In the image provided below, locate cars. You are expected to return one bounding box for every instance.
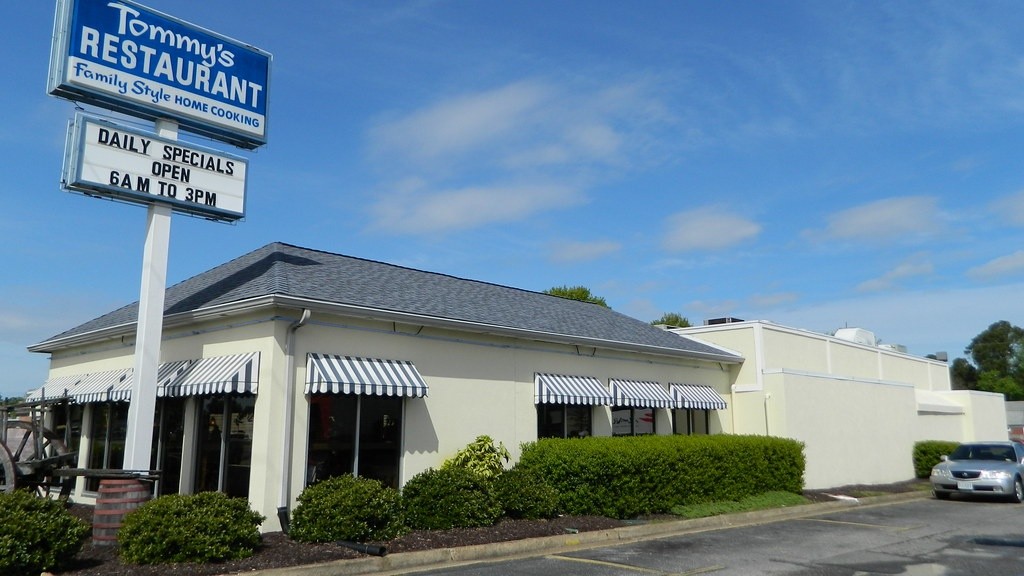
[929,441,1024,503]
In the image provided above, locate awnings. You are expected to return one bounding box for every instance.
[25,374,88,404]
[304,352,429,397]
[165,351,259,397]
[109,358,193,400]
[608,378,676,408]
[60,368,133,405]
[668,383,728,410]
[534,372,614,406]
